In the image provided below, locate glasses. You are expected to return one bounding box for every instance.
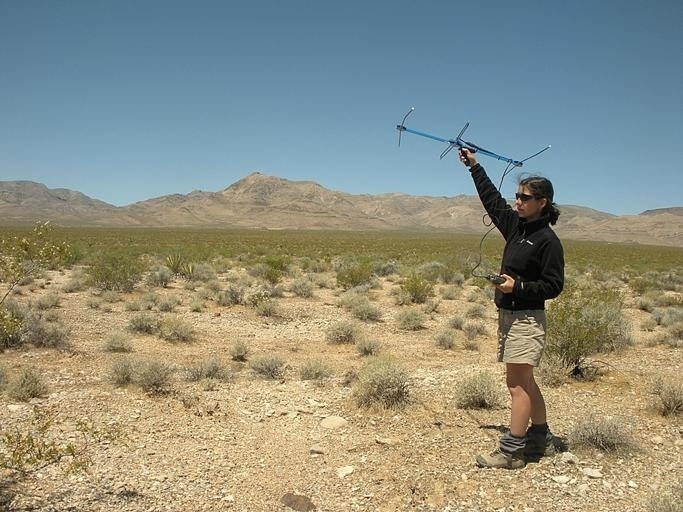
[517,193,535,202]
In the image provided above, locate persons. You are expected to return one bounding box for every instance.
[457,147,566,471]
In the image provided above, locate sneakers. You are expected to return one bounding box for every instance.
[523,432,555,455]
[477,447,525,469]
